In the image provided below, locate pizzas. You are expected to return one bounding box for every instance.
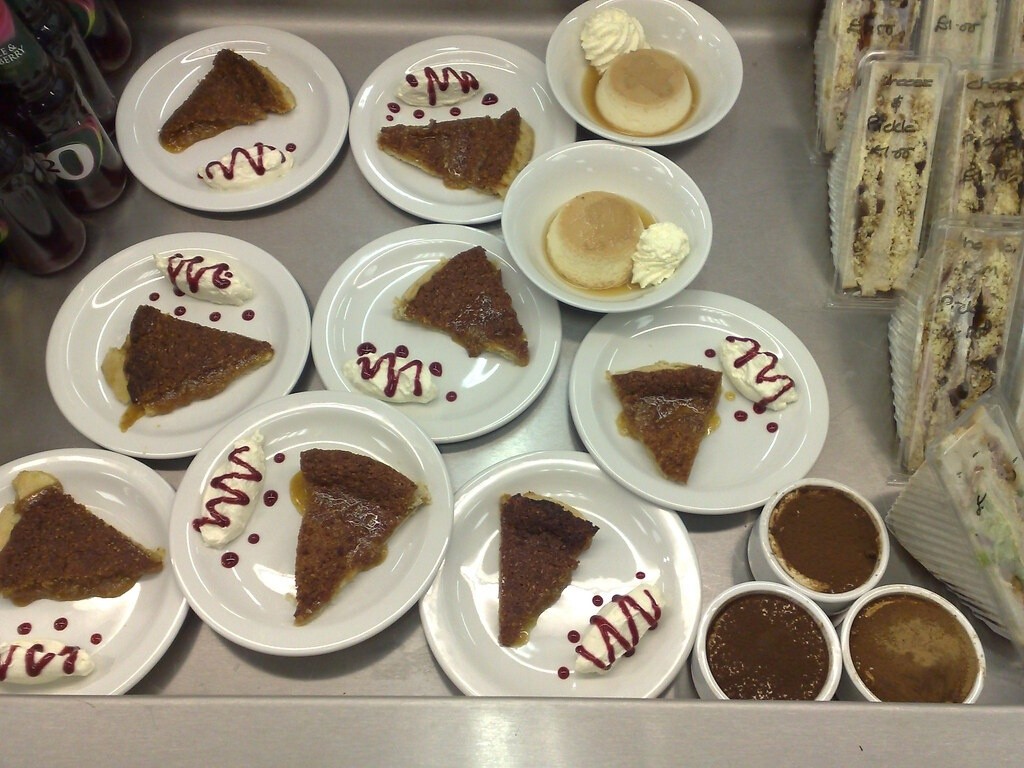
[605,359,724,487]
[494,491,601,648]
[376,106,537,200]
[101,304,274,417]
[159,50,298,154]
[292,448,432,626]
[0,469,166,604]
[394,243,529,366]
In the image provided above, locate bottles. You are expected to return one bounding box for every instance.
[0,0,132,277]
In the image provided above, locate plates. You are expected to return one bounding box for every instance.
[43,229,312,461]
[418,449,704,700]
[349,34,577,225]
[114,23,350,214]
[567,289,830,517]
[168,389,456,657]
[0,447,191,697]
[311,222,563,447]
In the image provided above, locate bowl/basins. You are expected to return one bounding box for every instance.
[744,476,891,627]
[499,139,714,315]
[544,1,745,147]
[689,578,842,704]
[841,583,987,705]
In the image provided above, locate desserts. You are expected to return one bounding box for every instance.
[546,189,690,290]
[580,6,693,136]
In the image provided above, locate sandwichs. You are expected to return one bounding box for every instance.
[812,0,1024,653]
